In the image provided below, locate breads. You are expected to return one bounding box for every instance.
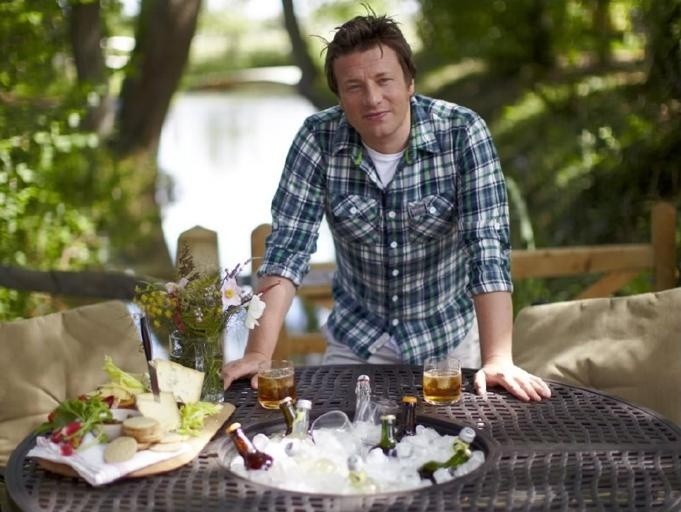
[134,359,206,430]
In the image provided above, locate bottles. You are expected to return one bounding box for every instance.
[225,423,274,471]
[354,374,480,493]
[284,442,336,482]
[279,395,312,442]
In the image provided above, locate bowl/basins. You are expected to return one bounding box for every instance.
[75,406,144,442]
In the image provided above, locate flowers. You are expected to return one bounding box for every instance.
[129,239,282,398]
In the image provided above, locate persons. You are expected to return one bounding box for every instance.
[220,2,552,402]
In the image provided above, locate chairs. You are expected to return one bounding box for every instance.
[510,287,681,430]
[1,301,158,480]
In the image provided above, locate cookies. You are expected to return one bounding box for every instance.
[104,415,180,464]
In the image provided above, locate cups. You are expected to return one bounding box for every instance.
[422,356,464,406]
[257,358,297,411]
[310,410,363,454]
